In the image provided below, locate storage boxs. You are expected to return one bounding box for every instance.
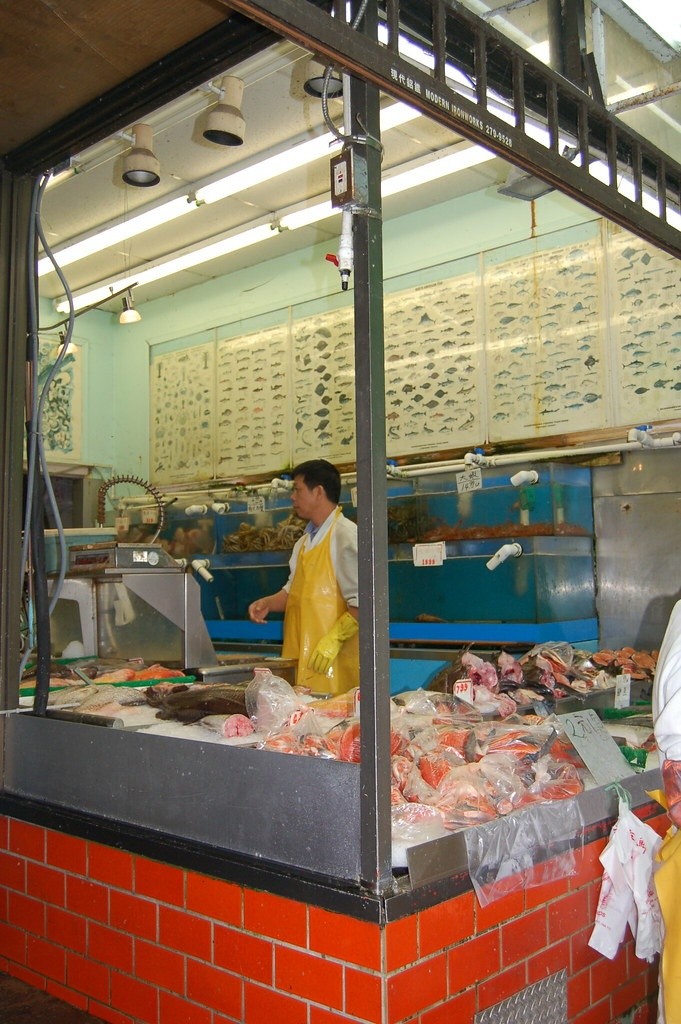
[44,528,118,574]
[152,462,600,648]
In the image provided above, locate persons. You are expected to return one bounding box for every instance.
[249,460,360,700]
[652,598,681,1024]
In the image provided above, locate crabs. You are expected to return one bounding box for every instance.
[222,513,307,551]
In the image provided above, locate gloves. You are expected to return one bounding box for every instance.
[307,611,359,675]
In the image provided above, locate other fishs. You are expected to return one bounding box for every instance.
[20,638,657,841]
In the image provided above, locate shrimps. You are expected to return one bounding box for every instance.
[422,516,588,542]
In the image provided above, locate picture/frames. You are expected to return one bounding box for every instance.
[22,337,84,460]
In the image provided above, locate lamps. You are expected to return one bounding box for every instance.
[114,124,160,187]
[57,322,78,355]
[53,140,497,314]
[498,145,599,202]
[119,289,141,324]
[331,0,681,232]
[37,98,422,276]
[303,54,343,98]
[199,76,247,145]
